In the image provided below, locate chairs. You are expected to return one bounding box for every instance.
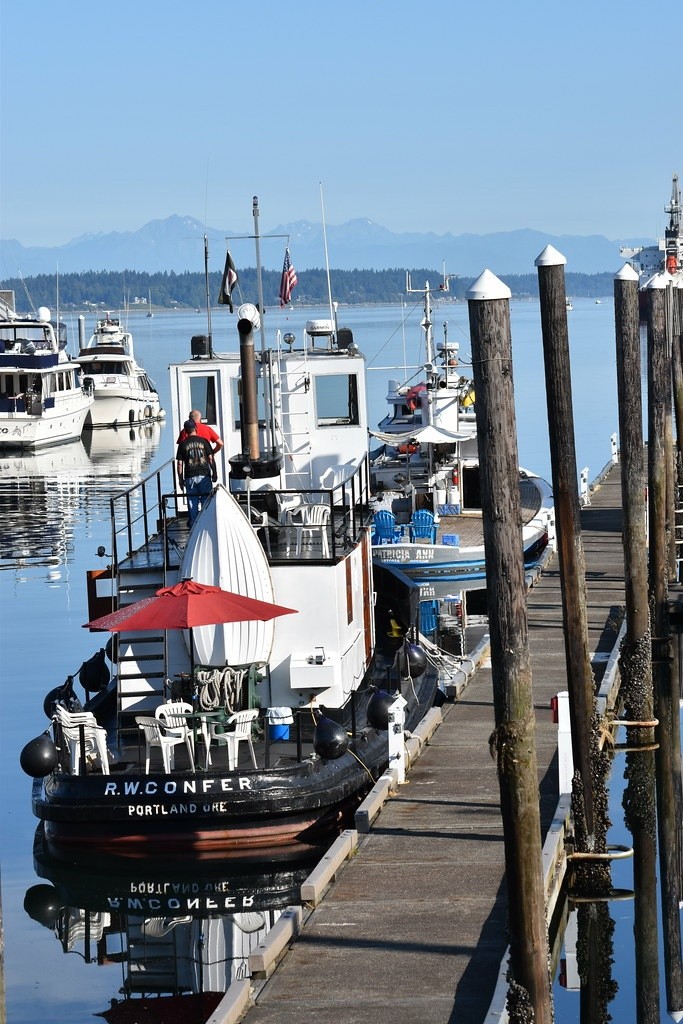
[56,704,111,775]
[285,503,331,559]
[205,708,258,772]
[155,702,213,769]
[319,463,359,521]
[240,504,273,559]
[407,508,440,544]
[135,716,196,774]
[419,600,437,635]
[266,483,314,538]
[370,509,405,545]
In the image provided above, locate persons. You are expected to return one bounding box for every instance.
[176,410,223,464]
[174,420,219,530]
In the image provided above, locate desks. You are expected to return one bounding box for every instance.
[167,711,220,772]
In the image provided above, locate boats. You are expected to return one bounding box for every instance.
[32,179,466,858]
[357,257,557,656]
[78,418,160,465]
[0,260,94,452]
[66,273,166,429]
[27,814,358,1024]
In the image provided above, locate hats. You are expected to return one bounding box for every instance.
[184,420,196,428]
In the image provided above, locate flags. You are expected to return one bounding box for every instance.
[278,248,297,308]
[217,250,239,313]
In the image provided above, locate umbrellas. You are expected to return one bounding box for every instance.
[81,576,299,767]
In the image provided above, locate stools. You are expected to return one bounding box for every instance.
[442,533,459,546]
